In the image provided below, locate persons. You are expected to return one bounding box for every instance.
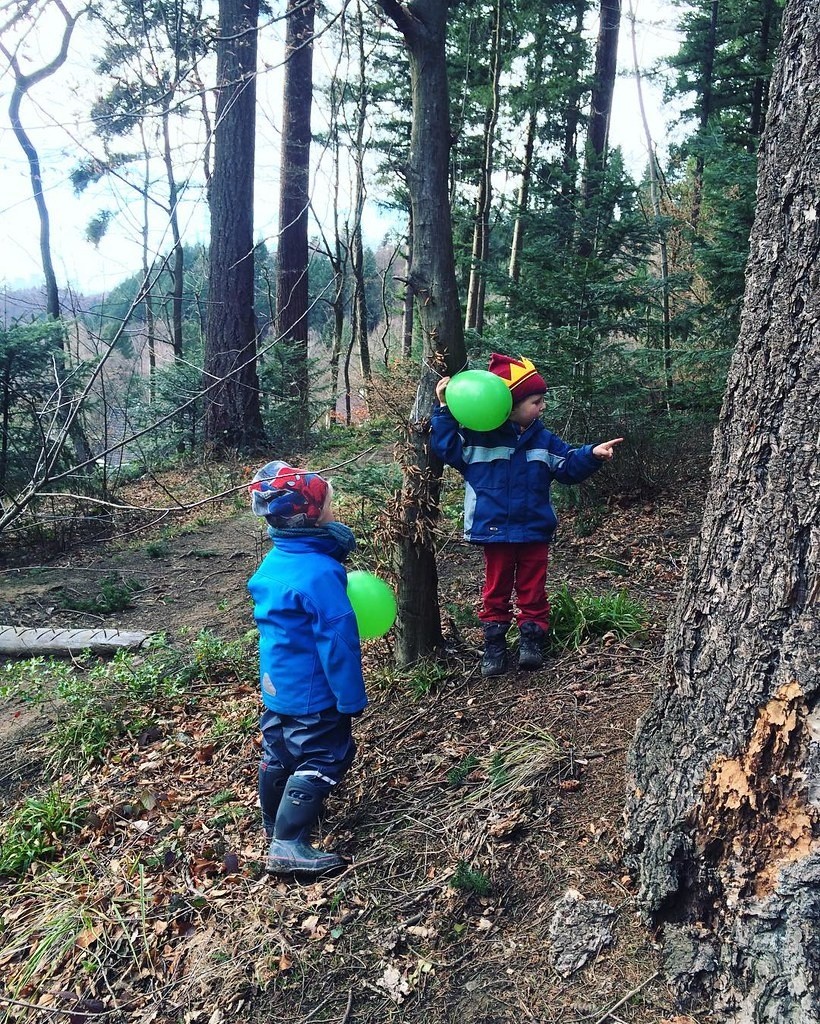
[429,353,624,677]
[247,460,368,874]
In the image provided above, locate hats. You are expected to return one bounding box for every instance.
[248,461,328,528]
[488,354,547,403]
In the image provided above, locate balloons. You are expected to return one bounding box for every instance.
[346,571,397,637]
[445,370,512,431]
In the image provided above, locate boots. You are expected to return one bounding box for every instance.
[481,622,511,676]
[264,775,348,877]
[259,761,292,844]
[518,621,545,669]
